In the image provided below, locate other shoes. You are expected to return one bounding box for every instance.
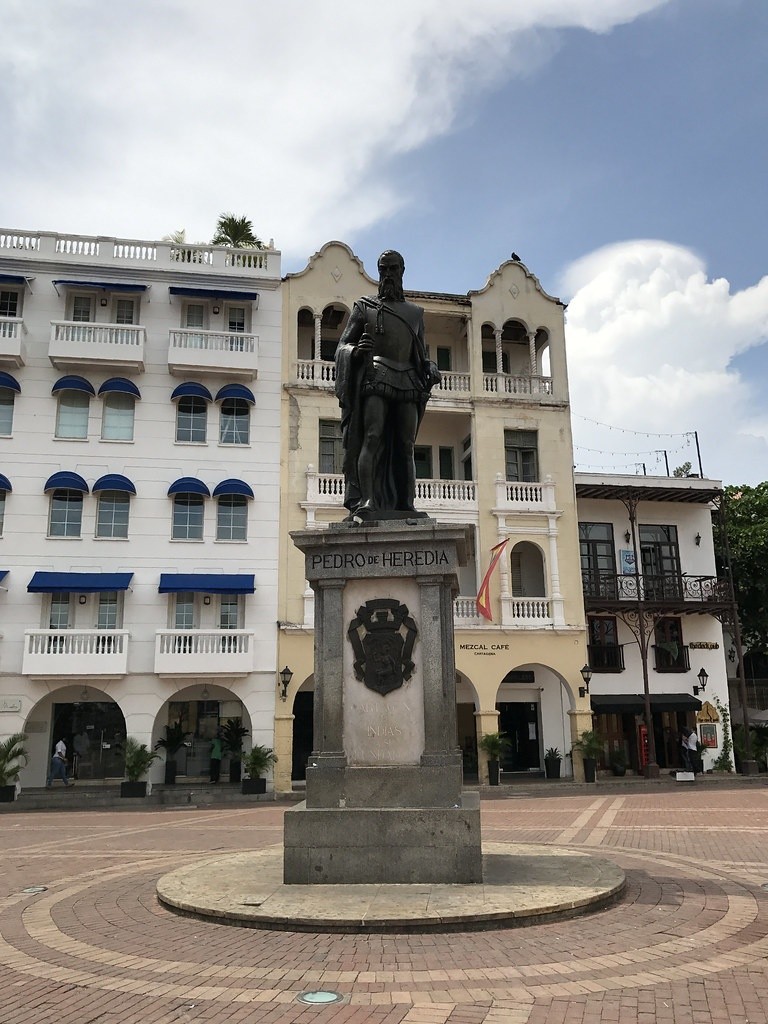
[47,782,51,789]
[65,783,75,787]
[208,779,216,784]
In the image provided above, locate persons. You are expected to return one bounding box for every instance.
[207,733,224,784]
[335,251,442,523]
[46,735,75,789]
[683,727,698,775]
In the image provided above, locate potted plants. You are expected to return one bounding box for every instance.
[480,731,513,784]
[611,750,627,776]
[118,738,164,798]
[156,721,192,784]
[0,731,30,801]
[239,744,278,794]
[571,727,606,782]
[544,747,563,778]
[217,716,252,782]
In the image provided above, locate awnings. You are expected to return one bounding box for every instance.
[157,573,256,594]
[0,371,21,393]
[27,571,134,592]
[590,693,702,714]
[170,381,256,405]
[0,273,35,295]
[168,286,260,311]
[51,374,141,400]
[167,476,256,499]
[0,571,9,591]
[44,471,137,495]
[0,473,12,492]
[51,279,152,304]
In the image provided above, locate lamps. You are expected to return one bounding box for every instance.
[202,684,209,698]
[696,533,701,545]
[81,686,89,700]
[101,299,107,306]
[79,595,86,604]
[625,529,631,543]
[213,307,219,314]
[279,665,293,697]
[579,664,593,697]
[204,596,210,604]
[693,667,709,695]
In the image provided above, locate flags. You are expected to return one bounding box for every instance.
[476,538,511,621]
